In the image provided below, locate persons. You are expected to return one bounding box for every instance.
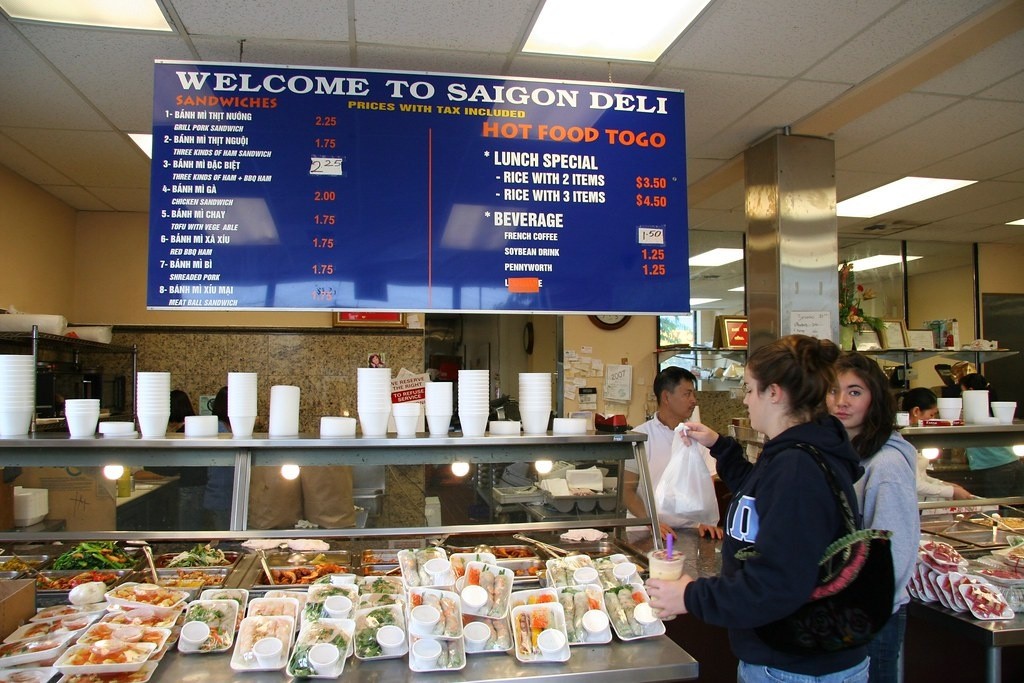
[368,354,385,368]
[645,335,867,683]
[622,366,723,541]
[959,373,1024,498]
[134,390,208,531]
[825,355,921,683]
[203,387,237,532]
[892,387,975,501]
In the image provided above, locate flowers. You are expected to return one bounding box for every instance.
[838,260,888,331]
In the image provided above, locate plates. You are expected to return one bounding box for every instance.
[908,539,1015,620]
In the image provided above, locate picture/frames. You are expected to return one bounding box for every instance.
[853,330,884,350]
[332,312,407,328]
[879,318,909,348]
[719,315,748,347]
[907,329,935,349]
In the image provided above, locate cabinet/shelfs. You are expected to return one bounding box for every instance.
[0,325,139,432]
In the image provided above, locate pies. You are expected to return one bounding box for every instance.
[401,547,646,668]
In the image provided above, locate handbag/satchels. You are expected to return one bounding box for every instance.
[654,423,720,526]
[733,445,895,652]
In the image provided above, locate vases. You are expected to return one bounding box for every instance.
[839,324,853,351]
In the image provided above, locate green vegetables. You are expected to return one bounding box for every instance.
[167,543,223,567]
[288,572,398,678]
[50,542,139,572]
[186,595,241,637]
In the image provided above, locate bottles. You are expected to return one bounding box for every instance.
[690,366,715,380]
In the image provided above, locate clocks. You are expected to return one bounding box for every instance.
[588,315,632,330]
[523,322,534,354]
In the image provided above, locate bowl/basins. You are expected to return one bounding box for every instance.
[0,355,36,436]
[137,373,171,438]
[227,372,257,439]
[519,373,552,434]
[937,390,1017,425]
[356,367,489,438]
[148,549,666,683]
[65,399,100,437]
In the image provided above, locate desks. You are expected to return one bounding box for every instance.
[115,475,180,530]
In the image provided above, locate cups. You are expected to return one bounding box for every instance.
[647,549,686,620]
